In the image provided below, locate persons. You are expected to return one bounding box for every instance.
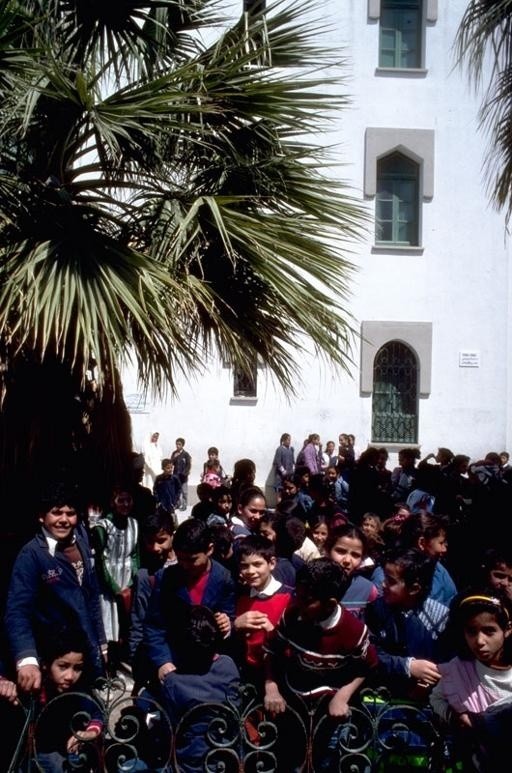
[7,488,108,691]
[265,434,354,505]
[204,447,222,476]
[91,459,457,772]
[355,444,511,509]
[429,557,511,728]
[140,431,164,494]
[171,438,191,510]
[0,642,106,771]
